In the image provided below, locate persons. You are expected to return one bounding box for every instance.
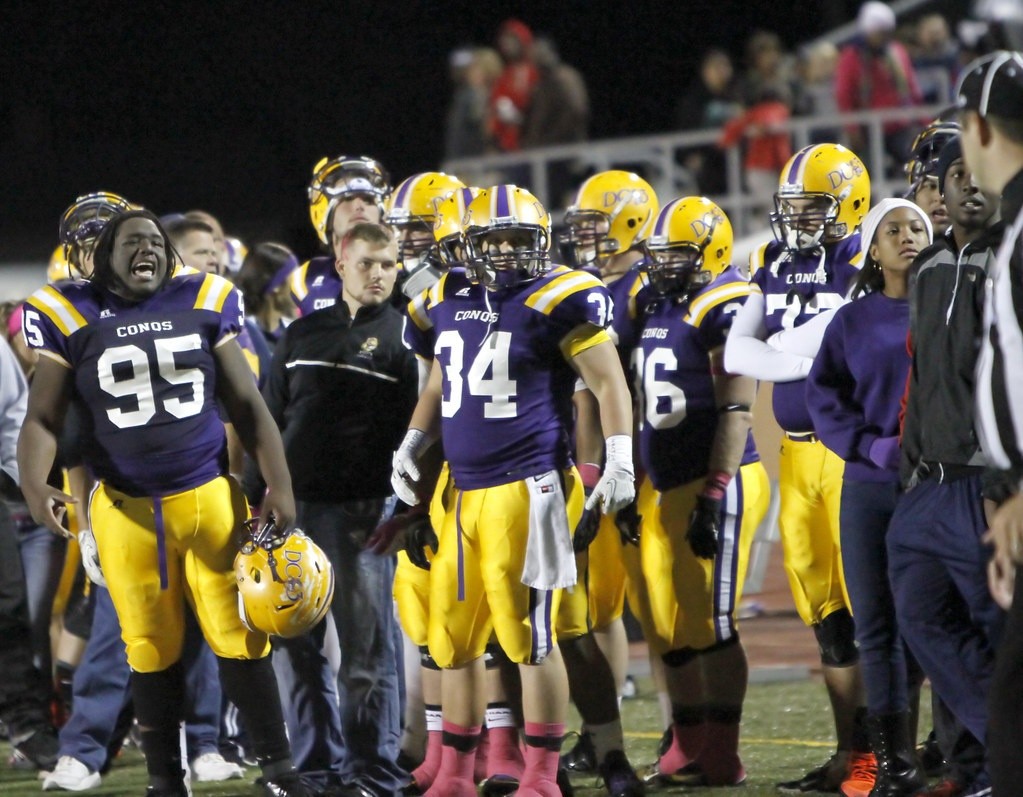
[391,185,636,796]
[621,194,760,788]
[0,0,1023,797]
[18,210,294,797]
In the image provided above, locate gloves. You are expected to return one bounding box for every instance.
[389,427,434,509]
[584,435,643,545]
[682,492,723,560]
[869,434,902,469]
[366,510,440,571]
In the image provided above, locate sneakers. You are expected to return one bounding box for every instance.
[598,748,648,797]
[774,749,880,797]
[634,724,749,791]
[189,753,245,782]
[42,756,102,791]
[558,730,606,790]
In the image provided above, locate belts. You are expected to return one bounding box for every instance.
[787,432,817,443]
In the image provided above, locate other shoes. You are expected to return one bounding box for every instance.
[257,770,308,797]
[6,730,42,771]
[318,782,373,797]
[915,741,992,797]
[146,769,194,797]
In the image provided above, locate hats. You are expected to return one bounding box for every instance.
[937,135,962,197]
[938,50,1023,120]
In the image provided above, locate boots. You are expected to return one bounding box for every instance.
[860,711,930,796]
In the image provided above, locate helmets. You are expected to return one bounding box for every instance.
[234,514,336,642]
[307,153,733,306]
[766,143,872,254]
[59,190,135,271]
[902,121,963,198]
